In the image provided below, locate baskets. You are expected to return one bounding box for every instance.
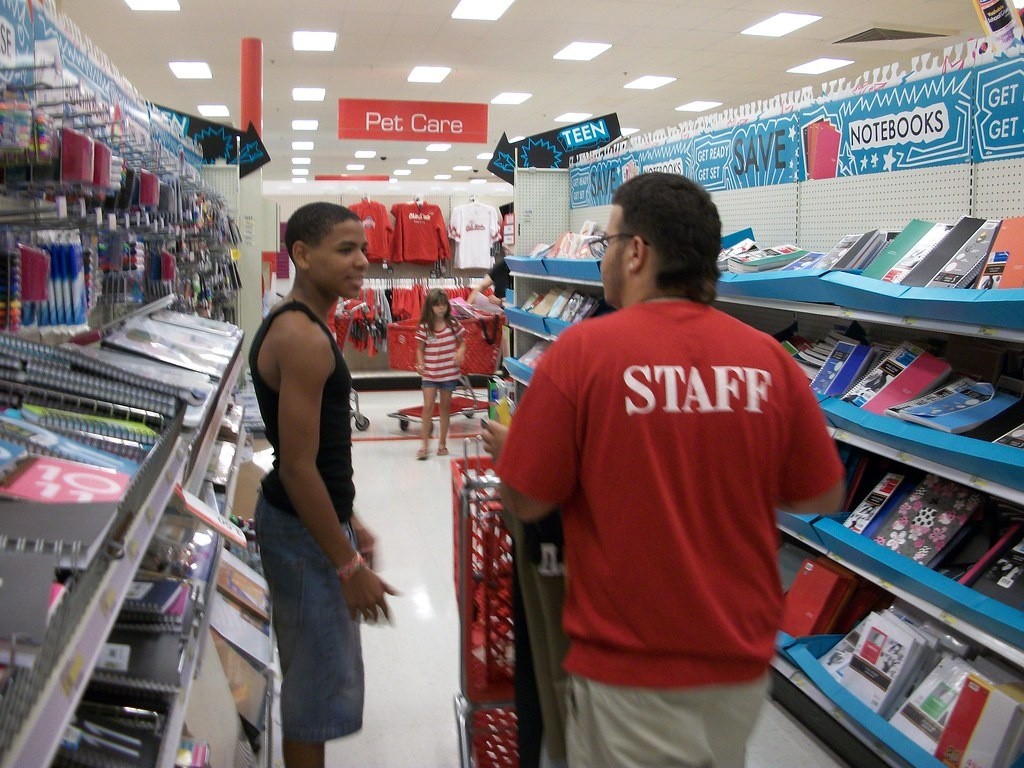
[388,313,504,374]
[449,457,512,704]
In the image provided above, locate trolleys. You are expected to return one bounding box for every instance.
[449,455,566,768]
[387,314,507,435]
[333,297,369,431]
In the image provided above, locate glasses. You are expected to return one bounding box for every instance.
[588,233,651,259]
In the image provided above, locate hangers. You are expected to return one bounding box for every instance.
[465,278,493,290]
[468,194,480,207]
[360,277,464,292]
[413,193,424,206]
[361,193,370,204]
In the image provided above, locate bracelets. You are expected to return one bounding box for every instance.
[336,553,363,578]
[418,364,424,365]
[501,299,502,306]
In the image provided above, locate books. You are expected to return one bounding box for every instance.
[0,352,214,470]
[779,321,1024,447]
[517,220,608,372]
[717,214,1024,290]
[52,580,194,768]
[0,501,117,669]
[773,456,1024,768]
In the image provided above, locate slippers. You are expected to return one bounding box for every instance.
[437,447,447,456]
[416,451,427,460]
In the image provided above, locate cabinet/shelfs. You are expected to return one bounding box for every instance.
[0,0,284,768]
[503,30,1024,768]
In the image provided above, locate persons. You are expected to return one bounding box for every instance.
[482,171,844,768]
[415,288,467,458]
[248,202,400,768]
[468,258,514,377]
